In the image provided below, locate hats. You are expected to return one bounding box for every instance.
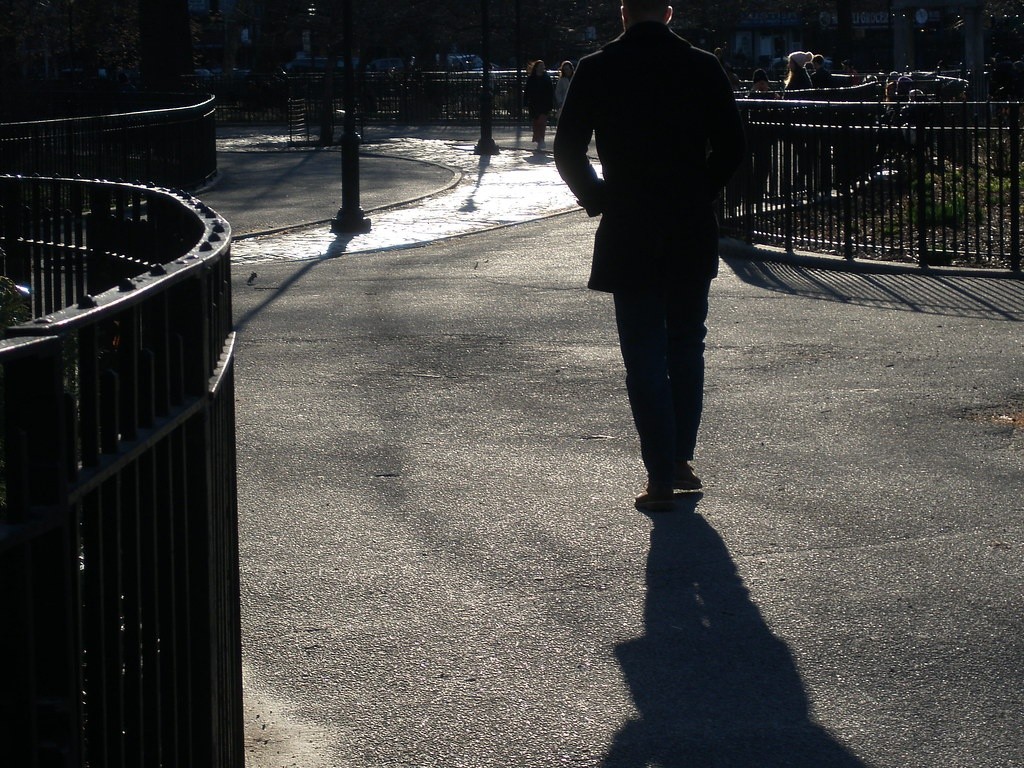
[753,69,768,83]
[791,52,812,68]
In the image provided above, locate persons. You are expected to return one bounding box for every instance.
[555,0,741,512]
[523,48,1024,153]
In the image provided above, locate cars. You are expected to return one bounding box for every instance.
[292,54,507,72]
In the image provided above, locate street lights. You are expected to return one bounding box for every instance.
[308,2,315,79]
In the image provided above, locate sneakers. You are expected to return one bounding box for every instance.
[636,493,675,508]
[672,465,703,490]
